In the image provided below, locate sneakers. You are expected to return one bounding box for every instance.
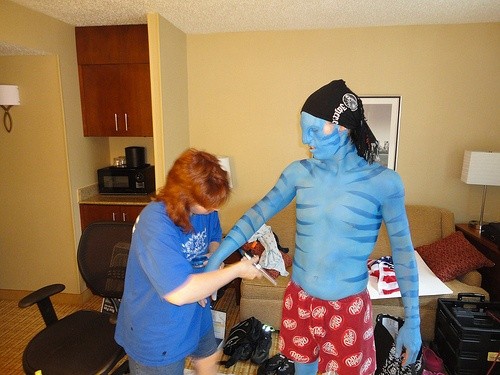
[238,334,272,365]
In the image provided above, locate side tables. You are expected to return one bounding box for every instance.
[456,223,500,324]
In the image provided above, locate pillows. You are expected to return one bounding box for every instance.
[242,242,292,279]
[414,229,494,283]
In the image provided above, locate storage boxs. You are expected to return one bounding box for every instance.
[433,292,500,375]
[210,309,227,351]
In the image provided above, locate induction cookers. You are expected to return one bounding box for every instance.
[97,166,155,196]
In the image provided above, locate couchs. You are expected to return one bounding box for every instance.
[242,199,489,343]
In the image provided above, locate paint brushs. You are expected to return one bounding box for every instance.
[239,248,278,286]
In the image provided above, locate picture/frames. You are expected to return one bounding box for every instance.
[312,95,403,173]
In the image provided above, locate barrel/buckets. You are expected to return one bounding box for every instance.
[124,145,145,168]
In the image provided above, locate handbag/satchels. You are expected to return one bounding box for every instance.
[373,313,424,375]
[218,316,264,369]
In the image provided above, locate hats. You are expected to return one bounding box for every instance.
[300,79,383,166]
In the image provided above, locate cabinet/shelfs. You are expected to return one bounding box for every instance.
[78,204,146,281]
[78,64,153,138]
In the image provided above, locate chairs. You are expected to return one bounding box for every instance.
[18,222,135,375]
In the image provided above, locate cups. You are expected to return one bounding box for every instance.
[112,156,126,168]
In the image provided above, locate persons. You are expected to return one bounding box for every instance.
[114,148,263,375]
[197,78,423,375]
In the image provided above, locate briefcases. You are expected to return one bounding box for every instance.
[433,292,500,375]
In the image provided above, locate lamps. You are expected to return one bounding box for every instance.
[0,85,21,133]
[215,156,232,189]
[460,150,500,230]
